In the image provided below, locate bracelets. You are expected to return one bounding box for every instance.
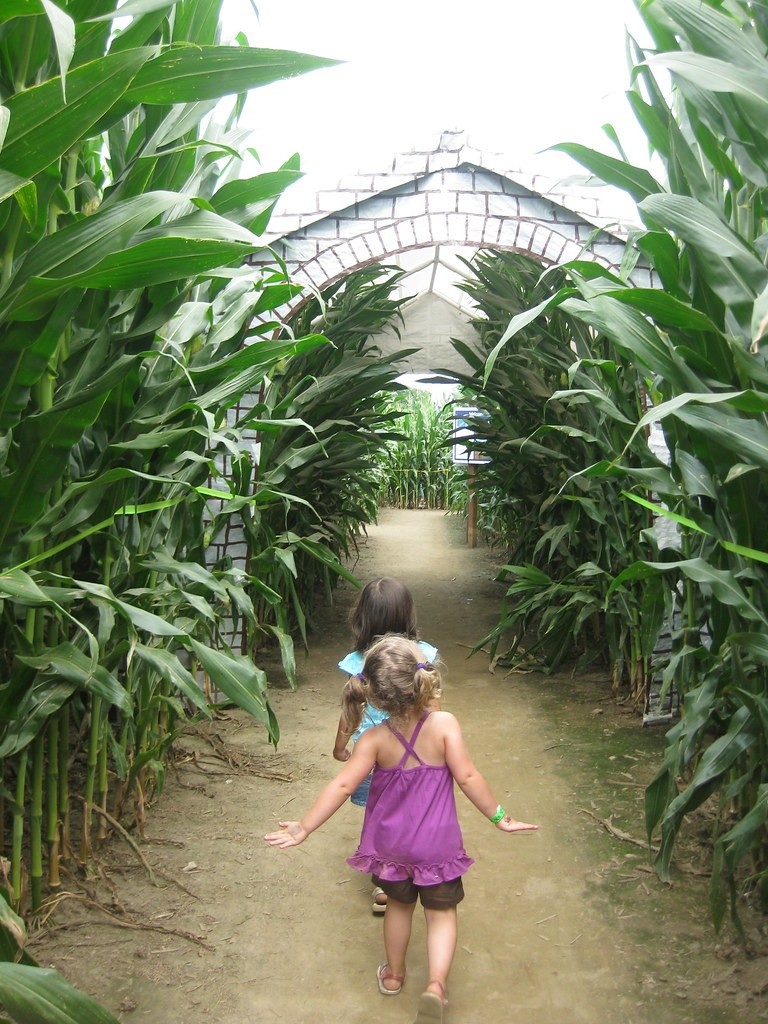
[490,806,506,823]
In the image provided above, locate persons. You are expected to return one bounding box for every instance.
[333,578,438,912]
[263,638,539,1024]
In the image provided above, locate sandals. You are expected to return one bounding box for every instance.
[413,980,448,1024]
[371,887,388,912]
[377,962,406,995]
